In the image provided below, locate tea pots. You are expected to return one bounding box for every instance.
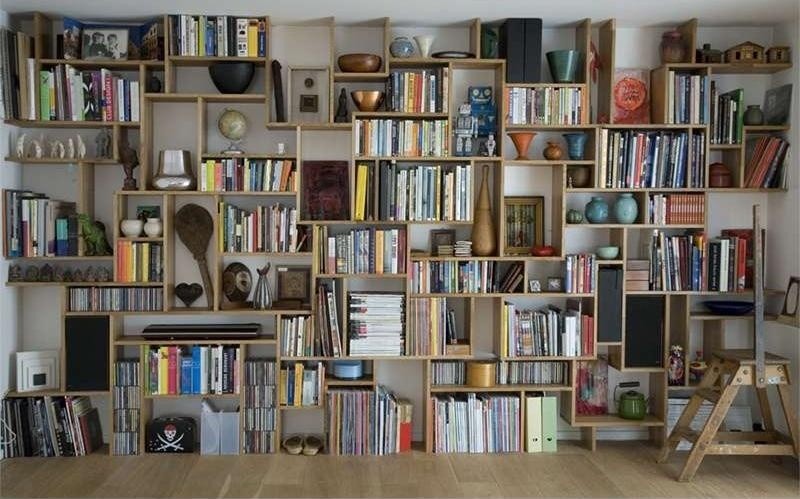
[613,380,656,420]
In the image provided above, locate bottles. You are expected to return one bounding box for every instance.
[253,262,274,311]
[743,104,763,125]
[389,37,411,58]
[144,218,162,239]
[658,32,686,63]
[586,196,609,224]
[542,142,561,160]
[612,193,640,224]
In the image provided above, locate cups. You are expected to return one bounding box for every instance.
[506,133,539,161]
[562,134,589,161]
[413,33,434,58]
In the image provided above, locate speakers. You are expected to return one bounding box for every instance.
[627,295,664,368]
[64,315,108,391]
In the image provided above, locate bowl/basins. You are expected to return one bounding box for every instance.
[209,62,255,95]
[120,220,144,238]
[596,246,620,260]
[337,54,382,72]
[351,91,386,112]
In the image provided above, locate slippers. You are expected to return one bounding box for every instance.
[282,434,323,456]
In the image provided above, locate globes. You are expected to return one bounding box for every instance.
[214,107,248,154]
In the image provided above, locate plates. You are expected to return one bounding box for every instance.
[702,300,754,316]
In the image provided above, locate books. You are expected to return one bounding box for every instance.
[502,19,794,452]
[316,66,519,456]
[2,13,324,452]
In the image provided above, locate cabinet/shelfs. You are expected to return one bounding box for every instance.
[0,9,793,456]
